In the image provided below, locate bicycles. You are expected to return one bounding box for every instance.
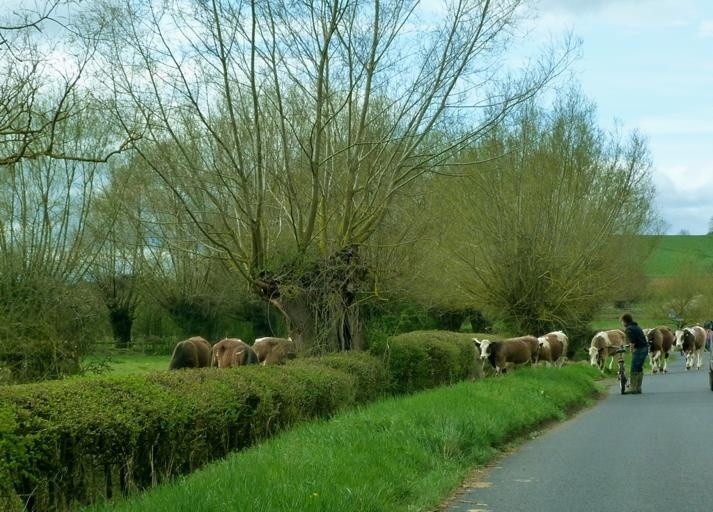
[606,343,630,394]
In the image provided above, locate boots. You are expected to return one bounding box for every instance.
[624,371,644,394]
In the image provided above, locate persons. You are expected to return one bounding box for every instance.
[705,328,713,392]
[619,313,648,394]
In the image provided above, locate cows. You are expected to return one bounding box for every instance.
[641,323,707,376]
[469,333,542,377]
[582,328,626,373]
[167,334,297,369]
[536,329,571,367]
